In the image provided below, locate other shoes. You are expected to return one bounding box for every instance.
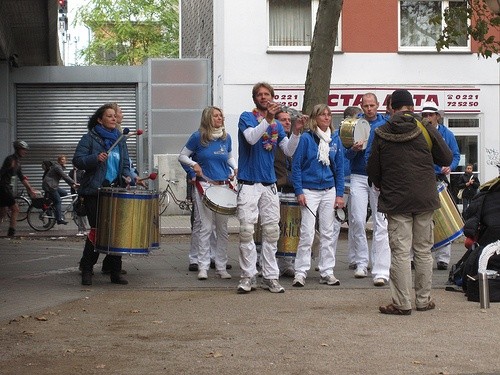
[79,262,94,286]
[7,227,16,239]
[101,266,128,285]
[43,222,54,228]
[57,219,69,224]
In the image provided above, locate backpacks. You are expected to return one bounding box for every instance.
[448,243,500,303]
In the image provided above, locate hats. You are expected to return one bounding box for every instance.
[418,101,444,119]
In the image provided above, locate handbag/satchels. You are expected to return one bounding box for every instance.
[457,188,465,200]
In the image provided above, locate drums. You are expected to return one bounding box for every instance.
[340,117,370,150]
[203,186,238,215]
[94,188,153,254]
[430,180,466,252]
[127,190,159,248]
[275,200,313,258]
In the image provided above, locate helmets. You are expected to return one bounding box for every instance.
[14,140,30,151]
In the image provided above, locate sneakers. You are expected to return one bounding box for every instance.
[416,302,435,311]
[260,276,285,293]
[349,264,370,270]
[197,269,208,280]
[379,304,411,315]
[292,275,305,286]
[319,274,340,285]
[189,263,198,271]
[373,278,386,286]
[210,261,232,270]
[437,261,448,270]
[354,268,368,278]
[237,277,258,294]
[215,270,232,279]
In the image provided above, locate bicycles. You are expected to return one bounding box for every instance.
[159,174,194,217]
[8,186,30,222]
[26,186,94,232]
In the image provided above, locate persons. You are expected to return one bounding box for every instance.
[42,161,53,228]
[72,104,131,285]
[457,164,480,212]
[411,102,461,270]
[257,112,295,278]
[180,109,234,271]
[179,147,235,271]
[44,155,80,224]
[178,106,238,280]
[0,140,37,237]
[461,176,500,292]
[237,82,309,293]
[291,104,344,287]
[79,103,144,274]
[367,89,453,315]
[344,93,391,286]
[69,160,91,236]
[315,107,371,271]
[384,96,392,118]
